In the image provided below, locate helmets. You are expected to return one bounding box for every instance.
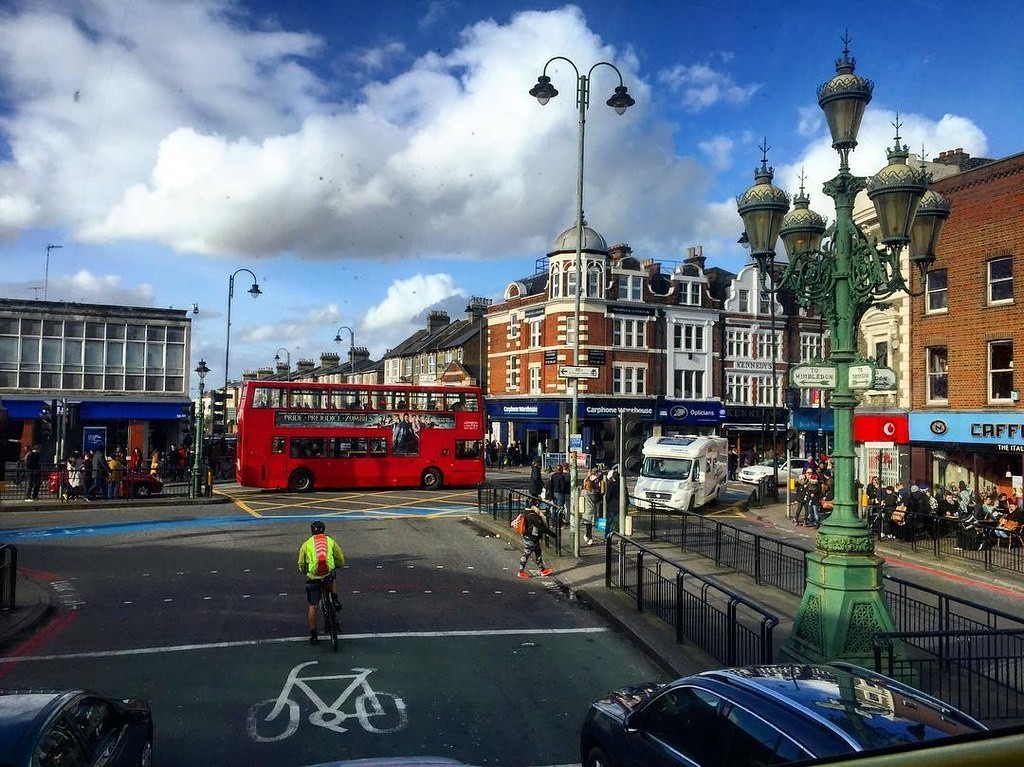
[311,521,325,533]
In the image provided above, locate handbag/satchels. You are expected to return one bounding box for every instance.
[820,498,834,508]
[578,497,585,514]
[624,515,632,535]
[892,503,907,520]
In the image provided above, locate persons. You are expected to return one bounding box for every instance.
[150,448,160,475]
[83,444,112,502]
[25,446,43,502]
[63,446,142,500]
[793,455,835,530]
[529,460,545,504]
[589,440,597,469]
[864,475,941,543]
[550,463,571,529]
[377,414,439,454]
[482,438,521,468]
[579,467,630,545]
[516,500,559,578]
[168,444,196,484]
[941,480,1024,550]
[298,521,345,645]
[728,449,739,481]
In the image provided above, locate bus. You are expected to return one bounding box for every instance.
[235,380,486,493]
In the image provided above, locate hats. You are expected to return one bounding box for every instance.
[912,485,918,493]
[921,484,928,491]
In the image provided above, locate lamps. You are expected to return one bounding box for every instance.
[1005,463,1012,478]
[957,461,963,473]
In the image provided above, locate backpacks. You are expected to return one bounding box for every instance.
[541,476,553,500]
[517,512,535,534]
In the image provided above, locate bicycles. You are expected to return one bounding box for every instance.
[316,565,350,651]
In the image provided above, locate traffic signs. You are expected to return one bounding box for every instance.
[559,364,601,381]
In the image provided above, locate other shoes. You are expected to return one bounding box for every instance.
[954,546,963,551]
[333,601,342,610]
[584,533,589,542]
[803,523,815,527]
[25,498,35,502]
[979,543,984,551]
[63,494,67,501]
[793,518,798,526]
[310,636,318,644]
[587,539,592,545]
[83,497,92,503]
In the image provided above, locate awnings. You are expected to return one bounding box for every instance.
[3,400,188,418]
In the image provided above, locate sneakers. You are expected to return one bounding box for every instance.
[518,572,530,578]
[541,568,552,575]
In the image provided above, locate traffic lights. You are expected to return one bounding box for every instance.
[43,405,58,442]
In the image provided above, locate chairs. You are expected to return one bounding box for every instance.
[313,401,320,409]
[305,402,310,409]
[296,402,303,410]
[260,401,267,408]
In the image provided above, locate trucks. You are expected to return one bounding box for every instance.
[633,434,730,513]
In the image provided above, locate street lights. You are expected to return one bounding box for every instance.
[193,358,212,497]
[44,244,64,301]
[274,347,290,382]
[222,267,263,435]
[734,27,956,699]
[334,326,355,384]
[528,56,637,439]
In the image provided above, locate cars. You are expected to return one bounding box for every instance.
[47,463,164,498]
[0,689,155,767]
[738,457,819,487]
[579,662,992,767]
